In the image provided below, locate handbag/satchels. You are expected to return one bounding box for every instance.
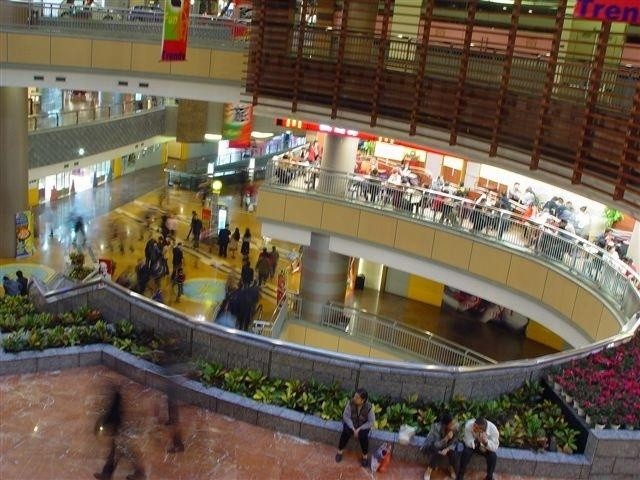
[378,440,391,474]
[370,445,387,474]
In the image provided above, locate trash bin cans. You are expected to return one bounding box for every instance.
[355,274,365,290]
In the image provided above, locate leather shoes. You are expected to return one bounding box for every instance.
[335,451,343,463]
[423,469,431,480]
[447,468,457,480]
[361,454,368,467]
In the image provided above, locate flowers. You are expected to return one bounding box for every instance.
[548,336,640,424]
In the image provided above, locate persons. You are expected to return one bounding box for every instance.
[165,212,178,244]
[429,176,512,232]
[359,161,419,213]
[60,0,78,17]
[418,413,461,480]
[86,381,147,479]
[595,227,627,271]
[158,334,195,455]
[80,0,94,19]
[162,215,169,240]
[217,224,231,257]
[191,217,202,248]
[241,227,251,257]
[282,139,321,187]
[454,416,500,479]
[239,179,256,212]
[197,176,212,208]
[15,271,28,296]
[191,210,198,228]
[132,232,279,332]
[72,216,87,255]
[334,387,377,468]
[509,183,590,259]
[230,228,241,257]
[2,276,23,296]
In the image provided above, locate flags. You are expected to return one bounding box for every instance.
[160,0,191,61]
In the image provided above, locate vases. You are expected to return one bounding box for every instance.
[595,425,635,430]
